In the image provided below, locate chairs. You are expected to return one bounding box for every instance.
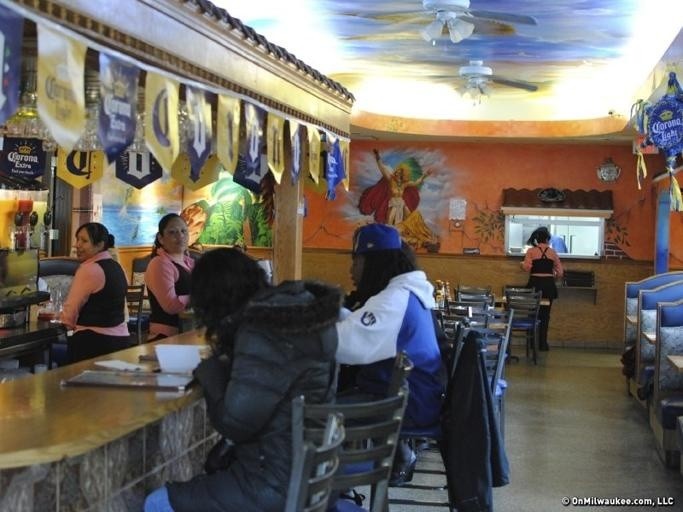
[126,281,152,345]
[49,343,69,369]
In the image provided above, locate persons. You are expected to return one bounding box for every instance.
[143,212,196,342]
[521,227,563,353]
[144,249,339,512]
[58,222,131,357]
[374,147,434,224]
[335,225,446,484]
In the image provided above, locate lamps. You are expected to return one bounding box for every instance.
[419,12,476,43]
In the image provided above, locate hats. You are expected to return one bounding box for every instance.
[353,224,401,252]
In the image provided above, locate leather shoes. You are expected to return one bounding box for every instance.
[388,449,416,486]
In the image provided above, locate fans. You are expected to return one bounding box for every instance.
[422,0,539,27]
[459,60,538,92]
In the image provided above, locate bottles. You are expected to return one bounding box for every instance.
[435,279,452,310]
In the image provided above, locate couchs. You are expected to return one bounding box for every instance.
[624,271,683,475]
[37,252,203,314]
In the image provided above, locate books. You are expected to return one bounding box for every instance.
[151,342,215,374]
